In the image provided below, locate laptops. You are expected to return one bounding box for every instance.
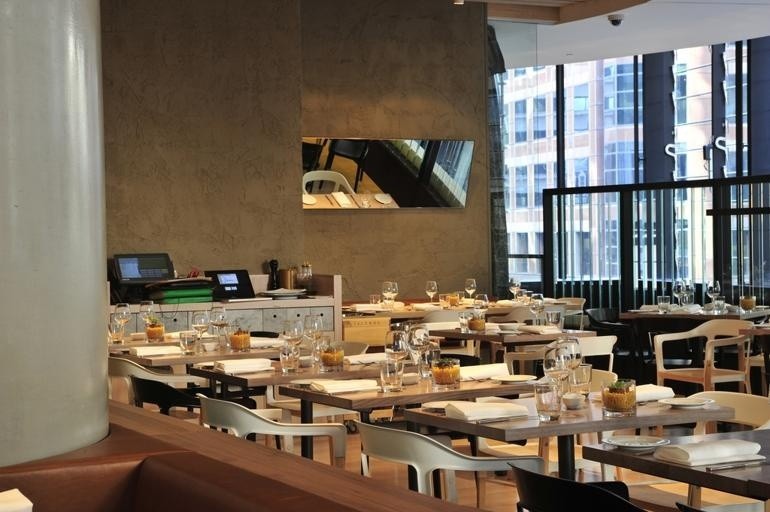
[203,269,272,303]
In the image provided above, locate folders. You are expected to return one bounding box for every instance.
[162,297,212,304]
[157,281,212,288]
[160,290,216,298]
[155,278,213,285]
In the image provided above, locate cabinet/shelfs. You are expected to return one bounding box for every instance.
[110,298,335,343]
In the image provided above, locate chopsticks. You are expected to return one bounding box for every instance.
[706,461,770,471]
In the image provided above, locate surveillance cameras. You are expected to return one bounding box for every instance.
[608,15,624,27]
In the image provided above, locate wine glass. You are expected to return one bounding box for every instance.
[282,320,302,345]
[302,315,322,370]
[509,277,520,299]
[426,280,436,303]
[209,308,228,354]
[385,330,407,360]
[578,349,583,351]
[673,280,685,311]
[408,325,428,382]
[543,343,571,392]
[530,293,543,327]
[465,279,477,297]
[707,280,720,312]
[558,339,581,369]
[190,310,209,356]
[381,282,393,306]
[474,294,489,318]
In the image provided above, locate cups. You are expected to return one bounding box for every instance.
[279,346,300,374]
[739,296,756,314]
[685,279,693,308]
[230,326,251,352]
[140,301,153,346]
[459,292,465,305]
[542,303,562,331]
[657,296,670,314]
[324,339,345,373]
[713,296,725,313]
[430,358,461,390]
[601,380,637,416]
[467,313,486,331]
[534,378,563,422]
[109,324,124,345]
[370,294,381,304]
[393,282,398,303]
[460,311,471,333]
[439,293,448,308]
[145,318,165,344]
[114,304,131,347]
[179,331,199,356]
[572,363,591,392]
[450,291,458,305]
[379,359,404,393]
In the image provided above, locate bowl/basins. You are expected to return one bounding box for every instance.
[727,305,739,311]
[130,333,146,340]
[564,394,585,409]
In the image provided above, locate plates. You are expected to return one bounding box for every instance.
[259,288,307,300]
[302,194,317,205]
[601,435,672,452]
[499,322,520,330]
[490,373,538,384]
[375,193,393,204]
[659,398,716,409]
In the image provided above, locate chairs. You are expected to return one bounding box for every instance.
[353,419,546,504]
[532,307,566,328]
[578,335,617,372]
[266,386,360,452]
[503,335,578,376]
[610,391,770,486]
[653,319,754,436]
[195,392,346,470]
[326,341,369,355]
[488,305,534,330]
[319,139,370,194]
[424,309,488,357]
[675,502,705,512]
[108,356,206,417]
[585,307,653,368]
[474,397,539,508]
[506,461,648,512]
[303,170,356,204]
[538,369,618,472]
[558,297,586,331]
[130,376,211,415]
[188,366,256,442]
[302,142,323,192]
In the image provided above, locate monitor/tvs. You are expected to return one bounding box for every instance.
[113,252,175,284]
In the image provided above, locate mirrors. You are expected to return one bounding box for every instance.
[301,136,475,211]
